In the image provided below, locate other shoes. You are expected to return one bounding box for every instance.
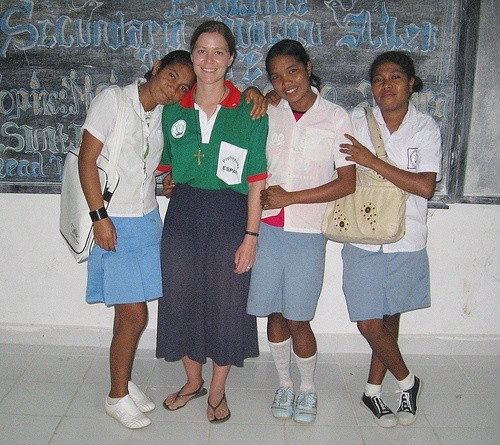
[127,380,155,413]
[104,393,152,429]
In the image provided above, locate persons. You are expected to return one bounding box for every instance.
[153,20,269,424]
[78,50,269,436]
[266,50,443,427]
[247,39,355,424]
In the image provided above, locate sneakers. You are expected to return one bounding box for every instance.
[271,385,296,419]
[394,375,424,426]
[360,391,398,428]
[293,389,318,424]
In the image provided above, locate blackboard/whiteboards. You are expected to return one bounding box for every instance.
[0,0,468,209]
[456,0,500,204]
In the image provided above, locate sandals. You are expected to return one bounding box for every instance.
[163,380,207,411]
[207,391,231,423]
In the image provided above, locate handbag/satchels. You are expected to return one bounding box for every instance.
[321,107,406,245]
[59,84,125,264]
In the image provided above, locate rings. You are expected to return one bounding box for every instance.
[248,267,250,269]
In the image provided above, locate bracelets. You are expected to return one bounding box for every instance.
[245,231,259,237]
[89,207,108,222]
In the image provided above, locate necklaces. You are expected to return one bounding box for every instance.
[194,87,226,118]
